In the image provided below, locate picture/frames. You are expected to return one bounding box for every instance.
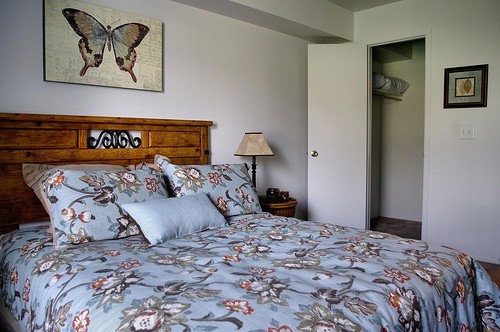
[443,63,488,110]
[43,0,164,92]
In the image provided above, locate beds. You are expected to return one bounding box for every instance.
[1,211,500,332]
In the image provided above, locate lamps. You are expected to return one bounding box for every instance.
[232,131,274,203]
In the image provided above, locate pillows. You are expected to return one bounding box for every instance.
[153,152,264,218]
[43,167,169,250]
[119,191,229,247]
[21,161,160,236]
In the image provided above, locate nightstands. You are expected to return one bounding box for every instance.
[257,192,298,220]
